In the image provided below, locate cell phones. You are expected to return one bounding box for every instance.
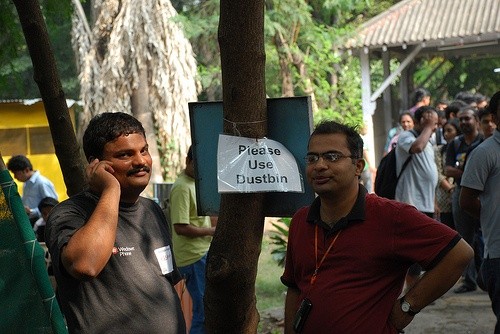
[293,298,312,333]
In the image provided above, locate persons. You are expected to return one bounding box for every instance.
[281,120,475,333]
[43,113,188,333]
[373,65,500,334]
[5,145,217,334]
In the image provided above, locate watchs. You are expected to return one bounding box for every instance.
[400,295,420,318]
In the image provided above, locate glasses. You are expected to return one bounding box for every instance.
[304,152,359,163]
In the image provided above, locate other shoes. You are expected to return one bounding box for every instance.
[455,285,476,293]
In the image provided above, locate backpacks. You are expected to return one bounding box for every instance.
[374,130,417,199]
[162,185,172,234]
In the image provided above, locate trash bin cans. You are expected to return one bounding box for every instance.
[152,184,173,228]
